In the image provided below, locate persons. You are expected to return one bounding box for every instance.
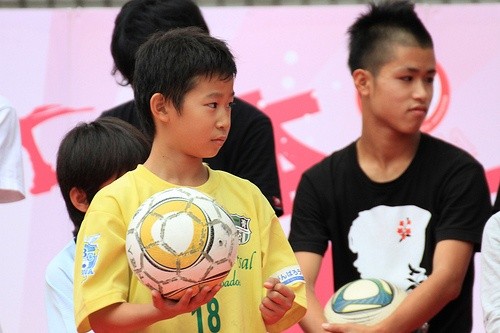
[0,96,26,204]
[288,7,492,333]
[480,210,500,333]
[44,117,151,333]
[74,27,307,333]
[99,1,284,217]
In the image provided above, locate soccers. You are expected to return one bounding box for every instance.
[124,186,241,301]
[323,277,406,333]
